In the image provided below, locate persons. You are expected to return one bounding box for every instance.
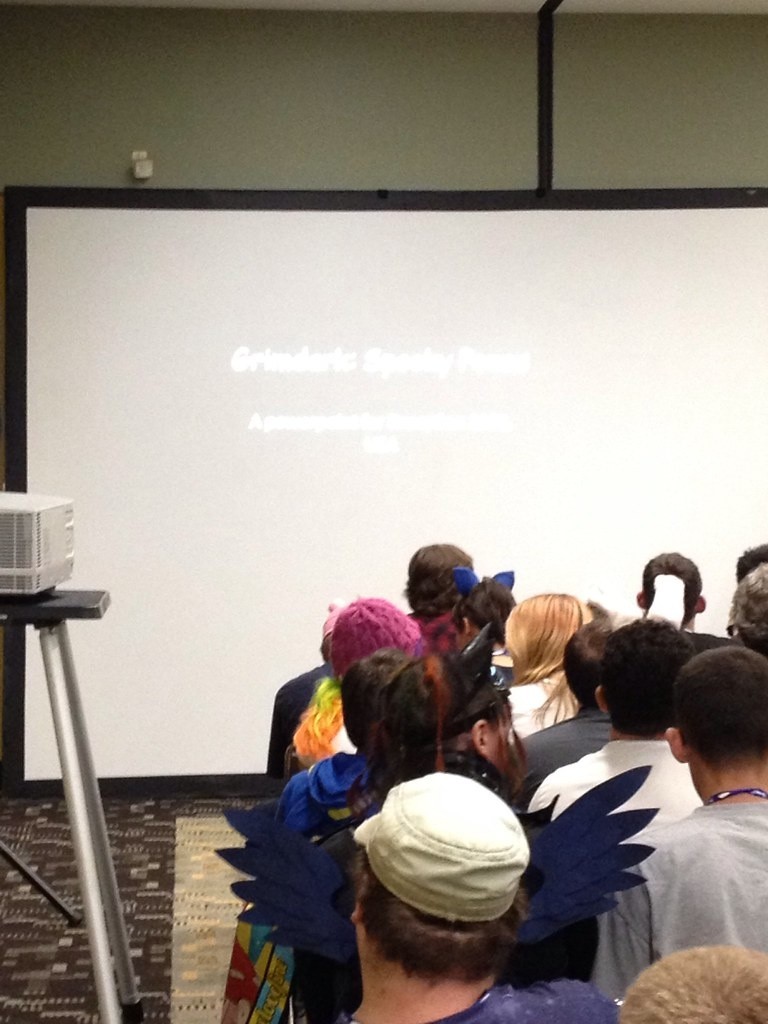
[263,544,768,1024]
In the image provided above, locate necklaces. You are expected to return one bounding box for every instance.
[704,788,768,806]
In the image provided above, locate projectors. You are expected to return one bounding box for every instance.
[0,491,75,596]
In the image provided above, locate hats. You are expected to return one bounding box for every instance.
[322,603,342,639]
[354,772,530,921]
[331,598,421,676]
